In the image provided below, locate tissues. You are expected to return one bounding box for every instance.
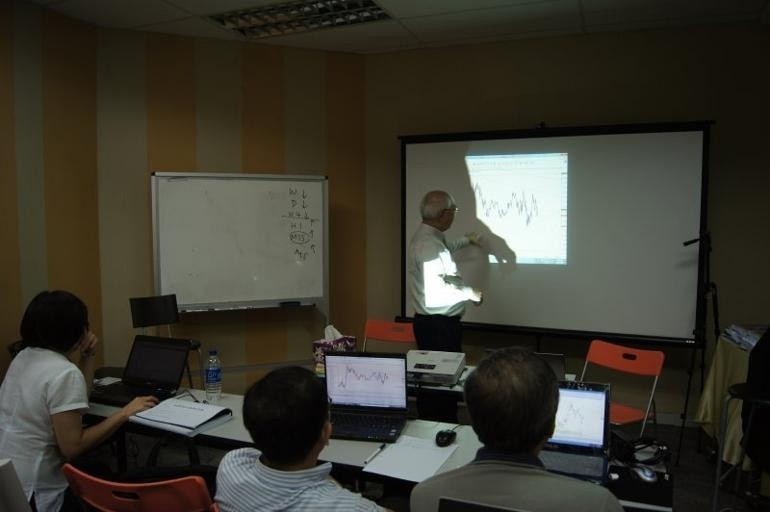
[313,325,357,362]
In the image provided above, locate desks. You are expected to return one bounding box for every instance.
[362,418,674,512]
[307,361,475,425]
[80,371,391,498]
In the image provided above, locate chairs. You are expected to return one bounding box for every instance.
[60,456,222,512]
[580,333,671,440]
[0,457,38,511]
[358,317,419,356]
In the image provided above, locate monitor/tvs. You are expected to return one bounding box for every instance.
[129,293,178,327]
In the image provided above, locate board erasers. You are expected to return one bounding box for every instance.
[280,302,300,306]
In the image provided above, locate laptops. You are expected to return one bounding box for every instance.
[323,353,408,444]
[537,380,610,484]
[490,346,568,379]
[405,349,465,385]
[87,334,190,407]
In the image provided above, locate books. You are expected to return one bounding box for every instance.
[128,397,234,440]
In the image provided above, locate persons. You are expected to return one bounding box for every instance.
[404,344,628,512]
[402,188,485,353]
[211,364,395,511]
[0,289,161,511]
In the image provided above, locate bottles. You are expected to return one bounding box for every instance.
[205,348,220,402]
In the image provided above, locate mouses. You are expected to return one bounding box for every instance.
[435,429,456,447]
[632,465,658,485]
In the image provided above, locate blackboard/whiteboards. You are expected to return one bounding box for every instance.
[151,172,330,312]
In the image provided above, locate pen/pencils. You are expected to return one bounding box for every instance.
[365,444,385,464]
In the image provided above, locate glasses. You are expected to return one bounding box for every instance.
[444,207,461,214]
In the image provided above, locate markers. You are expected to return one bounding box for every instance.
[181,306,253,312]
